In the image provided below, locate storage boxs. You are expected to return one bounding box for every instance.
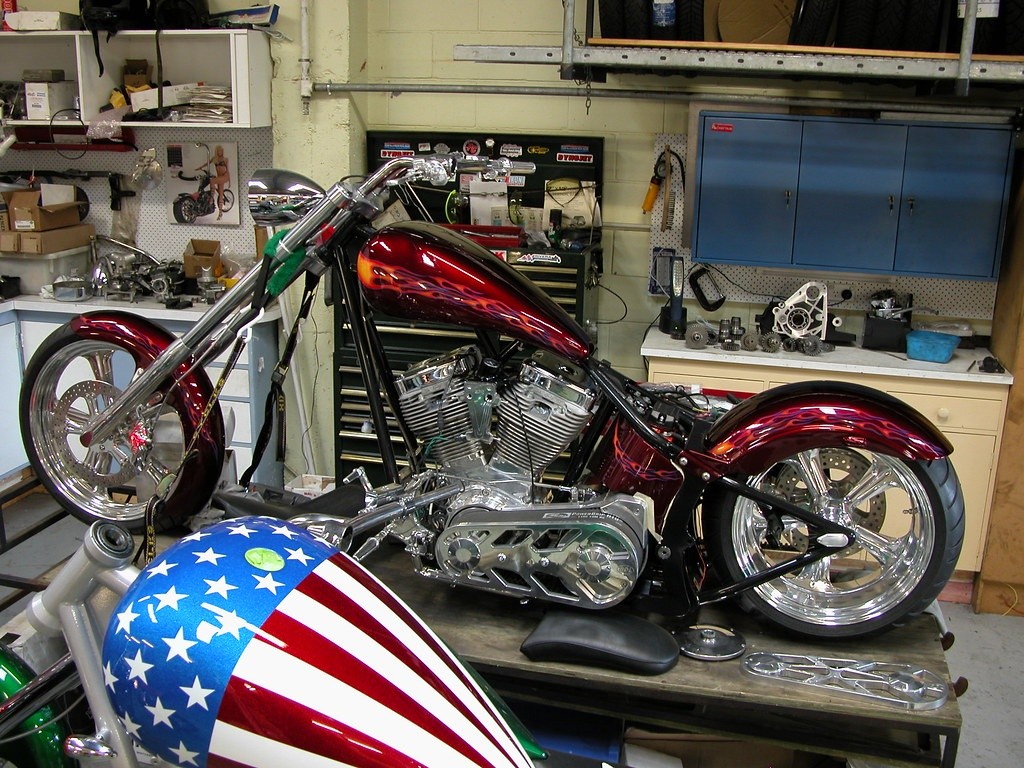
[181,237,223,280]
[0,186,97,297]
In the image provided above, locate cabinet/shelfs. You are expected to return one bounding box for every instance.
[0,290,287,505]
[0,27,273,129]
[555,0,1022,621]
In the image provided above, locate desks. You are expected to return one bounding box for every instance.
[365,533,963,768]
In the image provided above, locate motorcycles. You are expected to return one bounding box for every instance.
[18,153,966,656]
[167,166,235,223]
